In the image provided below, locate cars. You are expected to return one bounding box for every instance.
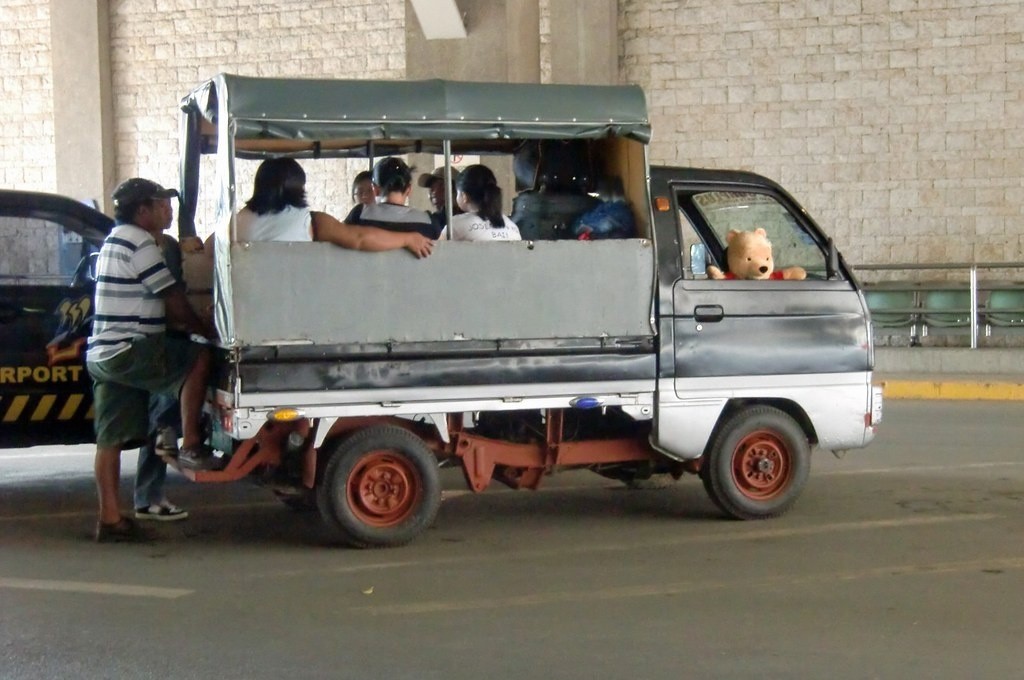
[0,188,152,453]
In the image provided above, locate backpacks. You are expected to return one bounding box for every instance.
[573,200,634,241]
[513,139,598,192]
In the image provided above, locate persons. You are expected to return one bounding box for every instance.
[205,157,436,260]
[418,166,465,231]
[342,157,434,241]
[86,179,217,542]
[351,171,376,205]
[435,164,522,240]
[134,184,190,519]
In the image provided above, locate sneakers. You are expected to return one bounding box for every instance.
[156,425,179,457]
[135,501,188,521]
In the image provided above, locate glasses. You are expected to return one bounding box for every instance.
[354,190,374,196]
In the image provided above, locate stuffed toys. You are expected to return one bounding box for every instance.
[706,228,807,280]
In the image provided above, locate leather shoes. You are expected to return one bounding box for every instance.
[178,445,216,469]
[97,518,159,543]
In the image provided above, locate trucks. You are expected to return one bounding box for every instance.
[163,73,888,549]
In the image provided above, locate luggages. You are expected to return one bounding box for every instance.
[507,192,592,241]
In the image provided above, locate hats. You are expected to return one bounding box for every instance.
[372,158,412,186]
[112,178,179,208]
[418,165,460,188]
